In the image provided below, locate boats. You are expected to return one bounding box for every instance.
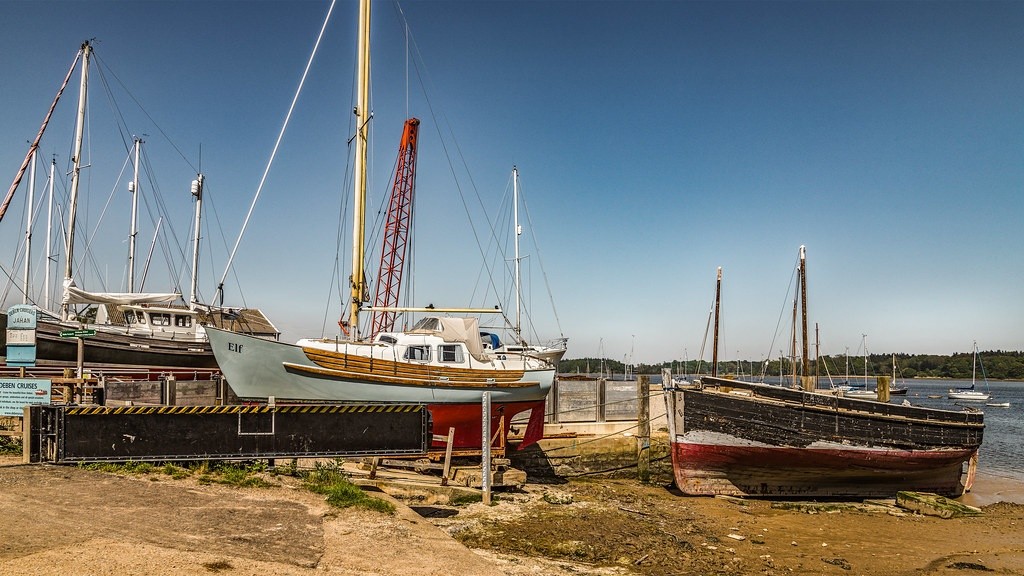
[928,395,943,398]
[659,245,988,501]
[986,403,1009,407]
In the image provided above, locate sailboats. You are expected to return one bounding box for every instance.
[947,339,992,400]
[460,165,569,366]
[200,0,559,407]
[0,39,277,370]
[830,333,908,403]
